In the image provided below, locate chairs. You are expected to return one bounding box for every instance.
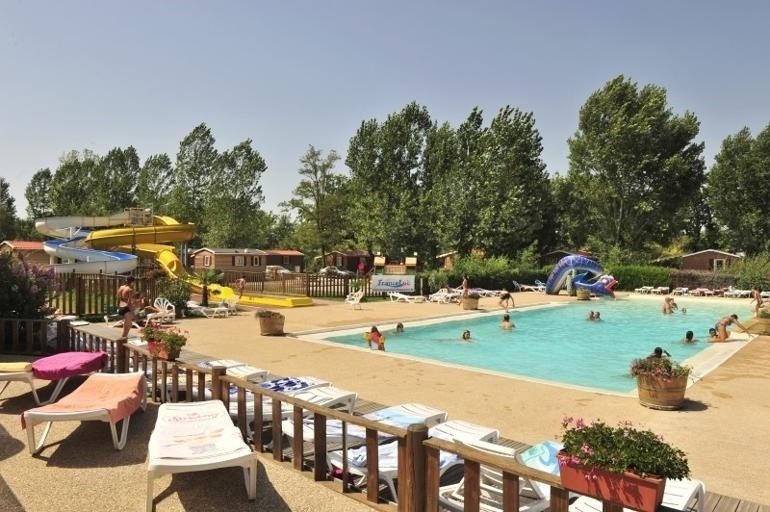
[187,300,208,317]
[154,297,176,324]
[211,300,229,319]
[569,469,709,512]
[535,279,548,290]
[429,288,449,303]
[345,291,364,312]
[513,281,523,290]
[438,435,585,512]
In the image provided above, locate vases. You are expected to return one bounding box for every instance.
[558,450,667,511]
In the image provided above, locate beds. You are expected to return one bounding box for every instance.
[0,350,108,407]
[275,401,448,456]
[147,364,273,390]
[202,374,329,402]
[388,290,426,303]
[17,371,147,453]
[230,386,357,435]
[149,359,239,372]
[144,397,259,512]
[328,419,509,501]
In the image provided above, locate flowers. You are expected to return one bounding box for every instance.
[556,416,693,478]
[630,358,694,383]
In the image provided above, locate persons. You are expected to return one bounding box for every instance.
[131,292,174,314]
[500,314,516,330]
[117,276,136,337]
[368,326,386,351]
[457,330,476,345]
[709,328,720,340]
[586,310,601,322]
[456,275,468,306]
[498,290,515,313]
[647,347,672,359]
[236,275,246,299]
[750,286,764,319]
[395,321,404,333]
[683,330,695,345]
[705,314,755,342]
[663,297,678,315]
